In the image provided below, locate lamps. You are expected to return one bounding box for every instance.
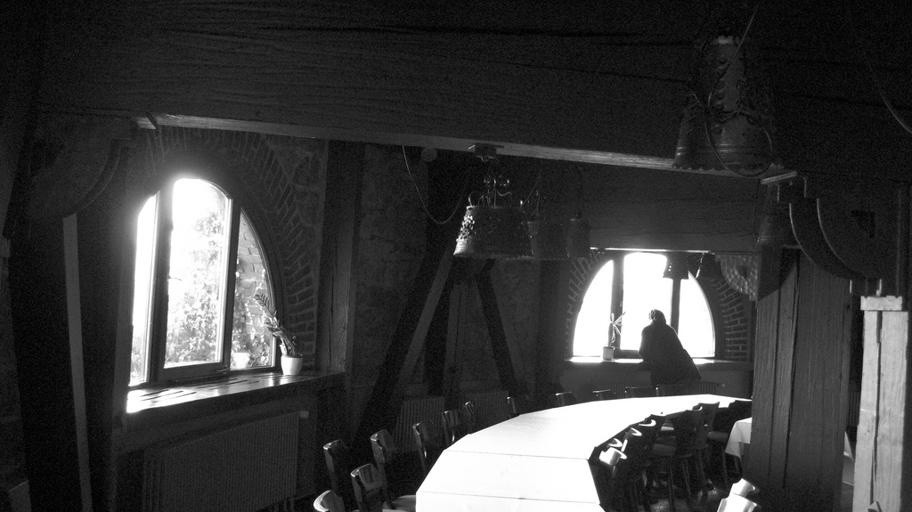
[453,158,589,261]
[672,21,781,171]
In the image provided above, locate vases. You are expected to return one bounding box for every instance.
[281,356,303,375]
[603,347,614,360]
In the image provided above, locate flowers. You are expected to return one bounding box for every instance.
[256,295,303,358]
[608,312,626,349]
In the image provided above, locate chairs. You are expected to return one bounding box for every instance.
[441,408,463,446]
[369,431,416,512]
[325,440,354,500]
[353,464,387,512]
[464,401,480,433]
[592,399,752,510]
[314,487,347,511]
[507,385,666,416]
[414,417,438,478]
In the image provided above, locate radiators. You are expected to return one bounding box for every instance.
[142,411,298,512]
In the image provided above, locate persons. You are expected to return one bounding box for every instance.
[639,309,701,396]
